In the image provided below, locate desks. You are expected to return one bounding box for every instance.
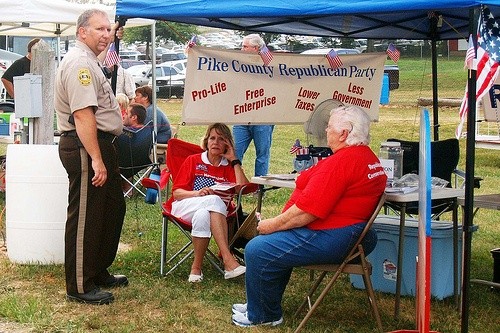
[249,173,468,322]
[457,194,500,315]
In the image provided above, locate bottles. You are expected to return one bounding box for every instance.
[378,141,404,189]
[145,164,161,205]
[21,129,28,144]
[14,131,22,143]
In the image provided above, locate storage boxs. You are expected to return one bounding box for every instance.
[348,215,462,300]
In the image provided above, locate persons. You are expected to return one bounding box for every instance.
[2,38,43,98]
[232,106,387,328]
[114,93,130,120]
[232,34,275,200]
[172,123,250,284]
[134,87,172,144]
[54,9,129,305]
[112,105,151,148]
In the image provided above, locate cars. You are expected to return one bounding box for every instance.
[119,29,432,99]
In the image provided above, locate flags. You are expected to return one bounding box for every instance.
[291,141,302,156]
[259,44,274,67]
[326,49,343,71]
[104,42,120,73]
[184,36,197,57]
[386,43,401,63]
[451,4,500,141]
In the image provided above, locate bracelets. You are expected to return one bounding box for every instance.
[231,159,242,167]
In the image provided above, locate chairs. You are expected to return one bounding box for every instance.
[116,118,154,198]
[142,138,258,279]
[383,138,483,221]
[153,124,178,164]
[288,192,385,333]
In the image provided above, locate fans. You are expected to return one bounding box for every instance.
[304,98,345,147]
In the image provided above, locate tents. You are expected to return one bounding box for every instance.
[110,0,500,333]
[0,0,158,169]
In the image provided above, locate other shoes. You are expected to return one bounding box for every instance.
[67,289,113,304]
[249,190,266,201]
[99,274,128,288]
[188,271,203,283]
[231,303,284,328]
[224,265,248,279]
